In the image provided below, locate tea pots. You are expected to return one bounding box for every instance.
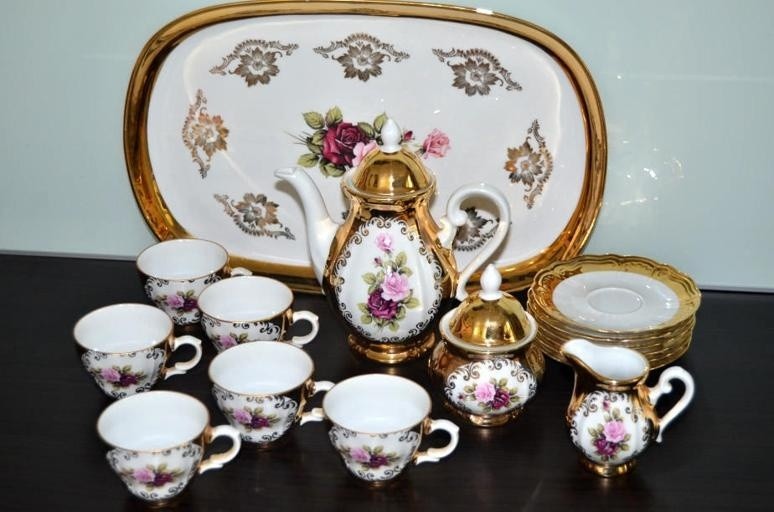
[272,115,514,368]
[557,335,697,480]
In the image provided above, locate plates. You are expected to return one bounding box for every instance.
[524,251,704,374]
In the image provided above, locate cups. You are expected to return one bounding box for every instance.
[94,388,244,511]
[134,236,256,328]
[206,339,340,448]
[193,272,321,358]
[71,300,205,404]
[320,369,463,489]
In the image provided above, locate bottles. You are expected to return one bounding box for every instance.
[424,260,548,433]
